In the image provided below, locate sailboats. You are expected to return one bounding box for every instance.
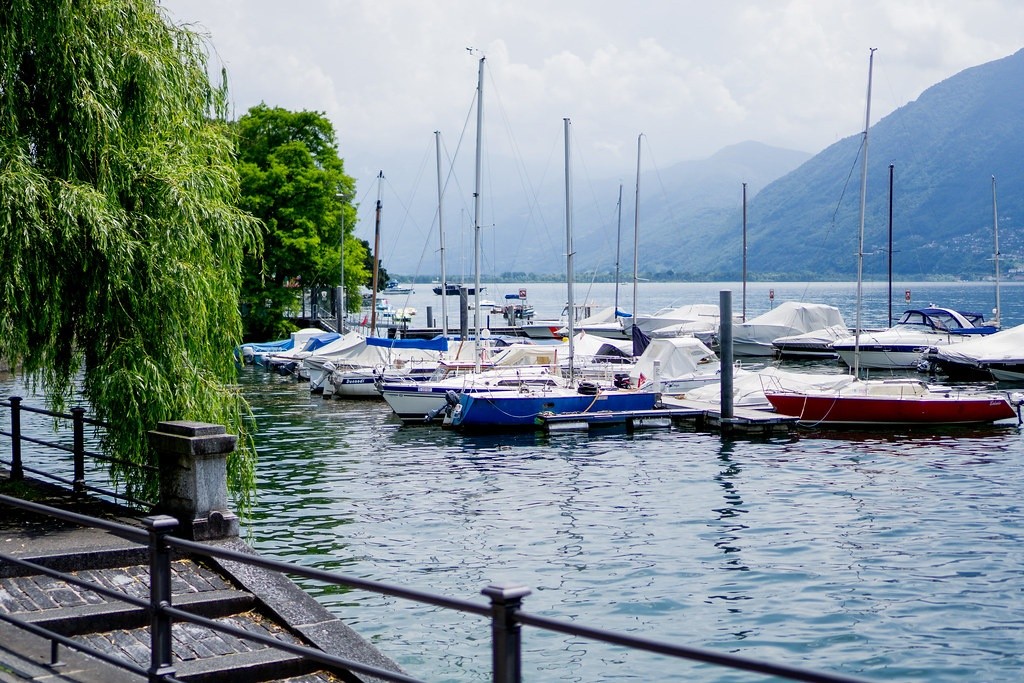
[236,44,1024,436]
